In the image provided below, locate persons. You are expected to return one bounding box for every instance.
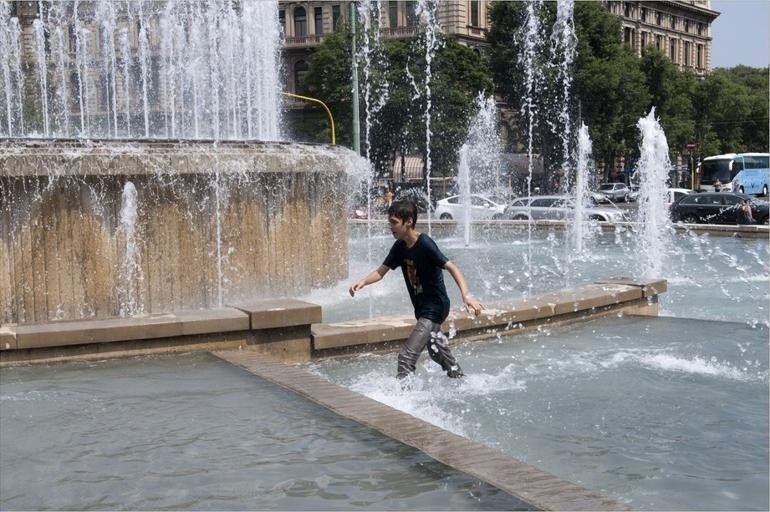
[384,188,393,211]
[349,200,486,380]
[713,179,722,192]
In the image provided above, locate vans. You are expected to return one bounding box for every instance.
[667,192,770,225]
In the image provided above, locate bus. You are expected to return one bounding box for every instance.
[696,153,770,198]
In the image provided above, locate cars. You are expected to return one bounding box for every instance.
[348,180,640,225]
[642,187,699,221]
